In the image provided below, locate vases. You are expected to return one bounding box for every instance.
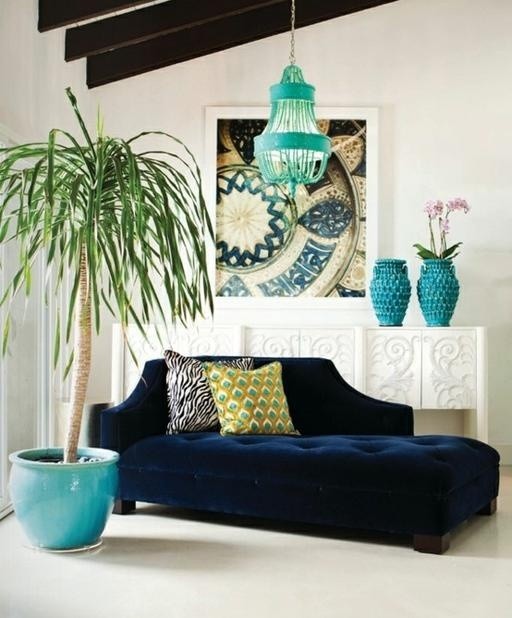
[370,258,413,326]
[417,261,463,329]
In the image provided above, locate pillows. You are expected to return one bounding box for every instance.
[162,350,251,433]
[205,361,301,437]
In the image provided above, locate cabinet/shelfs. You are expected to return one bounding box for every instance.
[111,324,487,446]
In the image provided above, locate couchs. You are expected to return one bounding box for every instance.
[100,357,499,553]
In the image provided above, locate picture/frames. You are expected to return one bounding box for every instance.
[203,105,381,313]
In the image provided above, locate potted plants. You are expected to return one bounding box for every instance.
[1,86,215,554]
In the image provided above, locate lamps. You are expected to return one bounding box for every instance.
[255,0,332,198]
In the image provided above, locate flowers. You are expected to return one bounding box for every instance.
[413,200,471,258]
